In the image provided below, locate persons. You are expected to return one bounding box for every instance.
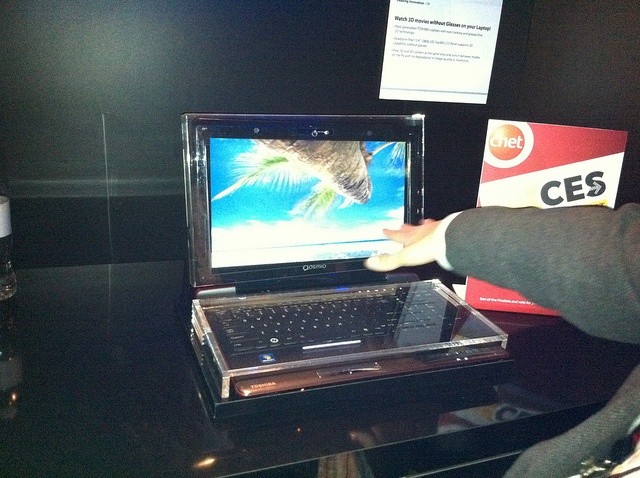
[362,200,640,478]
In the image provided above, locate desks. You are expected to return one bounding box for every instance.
[0,180,608,478]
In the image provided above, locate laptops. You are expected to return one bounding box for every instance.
[178,113,515,421]
